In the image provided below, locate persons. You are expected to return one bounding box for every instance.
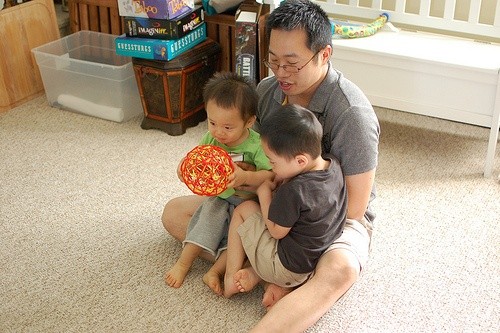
[161,0,381,333]
[163,69,278,298]
[222,101,350,298]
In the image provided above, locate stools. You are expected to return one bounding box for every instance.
[133,39,218,137]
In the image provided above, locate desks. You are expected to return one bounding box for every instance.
[0,0,63,112]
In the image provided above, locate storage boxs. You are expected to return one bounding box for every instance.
[115,23,207,61]
[30,30,143,123]
[118,0,194,20]
[235,1,260,94]
[123,5,205,39]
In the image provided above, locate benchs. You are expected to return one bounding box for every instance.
[259,0,500,178]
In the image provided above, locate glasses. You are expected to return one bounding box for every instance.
[263,47,324,73]
